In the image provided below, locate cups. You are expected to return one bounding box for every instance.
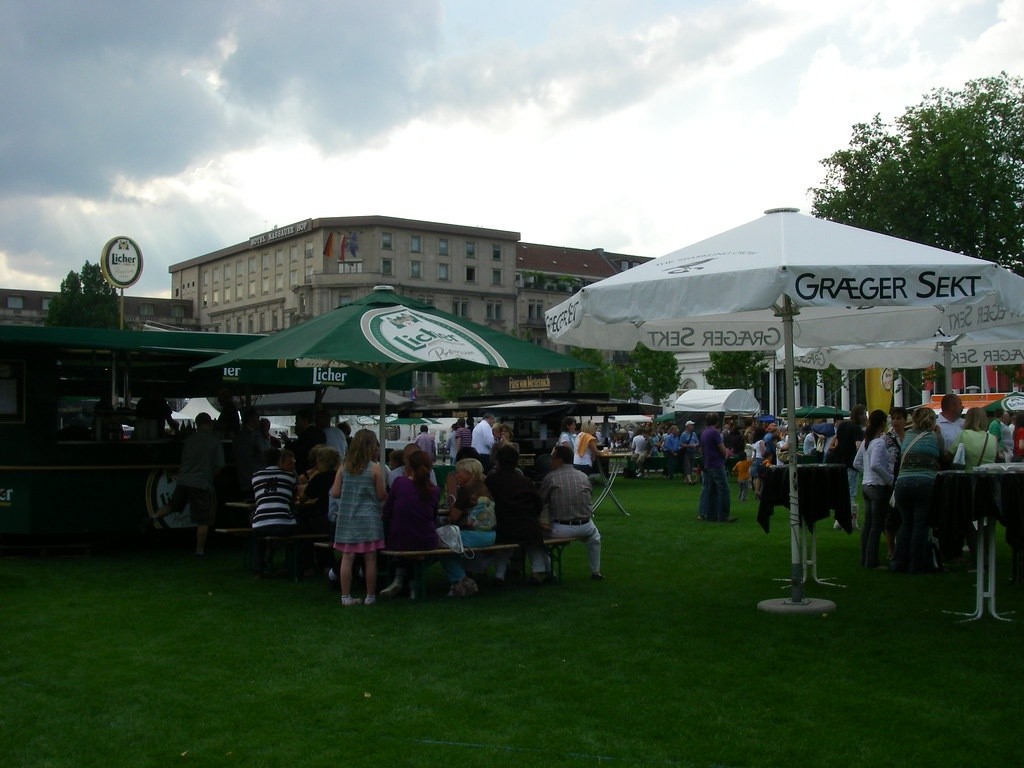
[604,447,609,455]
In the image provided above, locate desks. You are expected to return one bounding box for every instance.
[226,498,321,572]
[592,452,632,516]
[933,469,1024,625]
[518,454,536,472]
[756,463,853,590]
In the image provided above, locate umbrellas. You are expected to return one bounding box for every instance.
[250,388,411,416]
[779,405,850,425]
[340,415,441,442]
[982,392,1024,412]
[187,285,596,486]
[777,321,1024,396]
[654,412,675,422]
[544,208,1024,613]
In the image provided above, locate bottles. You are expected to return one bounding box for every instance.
[169,419,225,438]
[87,417,136,441]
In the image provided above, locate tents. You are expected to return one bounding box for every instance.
[674,389,761,426]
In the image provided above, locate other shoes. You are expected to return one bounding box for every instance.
[592,574,605,580]
[543,575,558,583]
[380,581,402,598]
[140,517,153,534]
[725,516,738,522]
[688,481,695,485]
[365,596,376,605]
[341,597,362,605]
[491,578,503,587]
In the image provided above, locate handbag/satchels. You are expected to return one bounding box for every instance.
[951,430,965,470]
[436,524,464,555]
[679,447,687,454]
[889,490,895,509]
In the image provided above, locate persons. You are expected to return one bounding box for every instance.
[559,418,678,480]
[853,394,1024,582]
[714,404,867,502]
[698,413,737,522]
[890,407,944,573]
[140,389,603,606]
[135,395,179,438]
[680,420,699,484]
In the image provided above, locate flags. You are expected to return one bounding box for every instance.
[339,234,345,258]
[351,232,358,257]
[323,232,334,256]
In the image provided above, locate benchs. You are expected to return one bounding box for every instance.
[625,455,739,480]
[214,527,586,604]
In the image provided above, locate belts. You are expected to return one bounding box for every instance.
[554,518,590,525]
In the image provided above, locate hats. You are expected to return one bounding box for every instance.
[685,420,695,426]
